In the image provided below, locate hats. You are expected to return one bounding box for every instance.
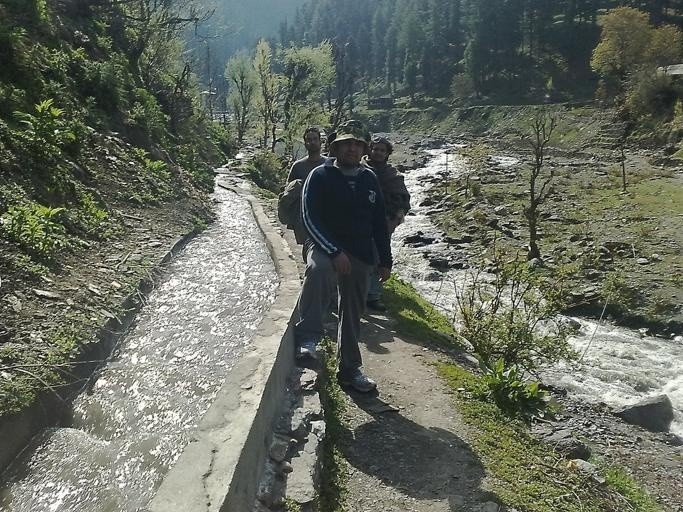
[329,120,371,145]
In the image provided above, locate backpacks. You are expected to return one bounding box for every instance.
[277,178,304,226]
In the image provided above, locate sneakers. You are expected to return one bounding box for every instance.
[339,370,375,392]
[296,342,316,360]
[367,300,386,310]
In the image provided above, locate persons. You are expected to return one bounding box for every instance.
[277,119,411,394]
